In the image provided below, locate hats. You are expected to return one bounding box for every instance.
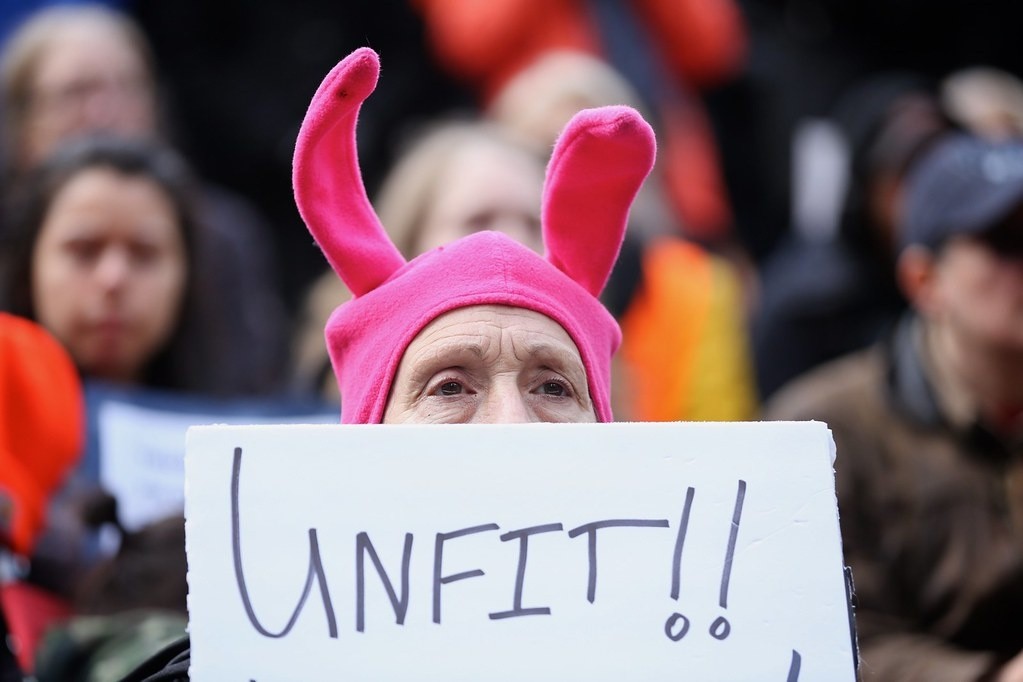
[0,312,84,558]
[291,47,656,424]
[898,136,1023,246]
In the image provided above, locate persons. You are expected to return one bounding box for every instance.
[123,47,657,682]
[0,0,1023,682]
